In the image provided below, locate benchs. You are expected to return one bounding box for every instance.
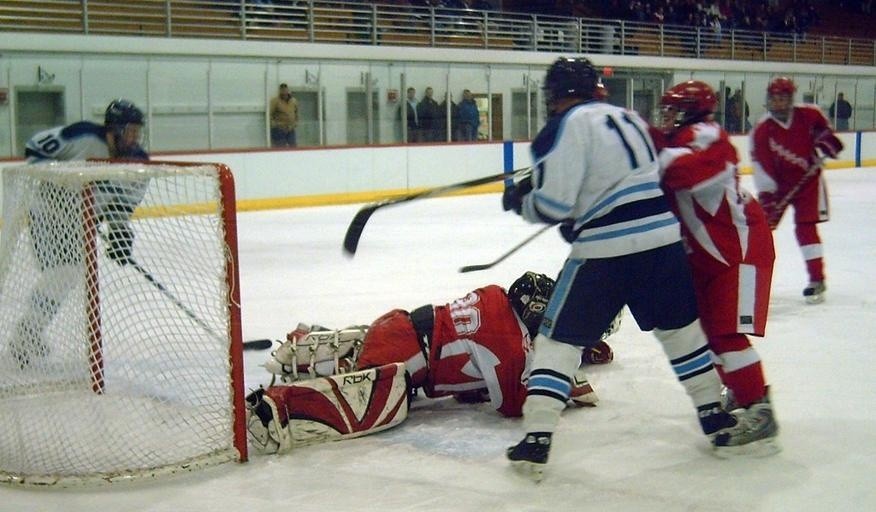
[0,0,876,67]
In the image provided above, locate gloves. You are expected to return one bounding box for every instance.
[107,228,135,268]
[502,175,533,214]
[815,131,845,159]
[758,193,781,228]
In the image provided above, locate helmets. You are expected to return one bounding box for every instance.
[542,55,599,97]
[507,270,556,339]
[104,98,143,126]
[767,77,797,95]
[660,80,718,114]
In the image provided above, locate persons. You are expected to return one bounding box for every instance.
[828,93,852,130]
[398,87,480,142]
[646,79,777,446]
[1,100,151,356]
[714,87,749,134]
[502,55,738,464]
[749,77,843,297]
[244,272,614,445]
[269,83,300,148]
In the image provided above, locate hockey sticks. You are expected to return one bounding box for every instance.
[93,226,272,351]
[459,223,557,272]
[344,166,528,255]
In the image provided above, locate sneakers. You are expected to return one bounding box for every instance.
[506,431,554,464]
[803,280,829,296]
[713,384,779,447]
[696,401,738,437]
